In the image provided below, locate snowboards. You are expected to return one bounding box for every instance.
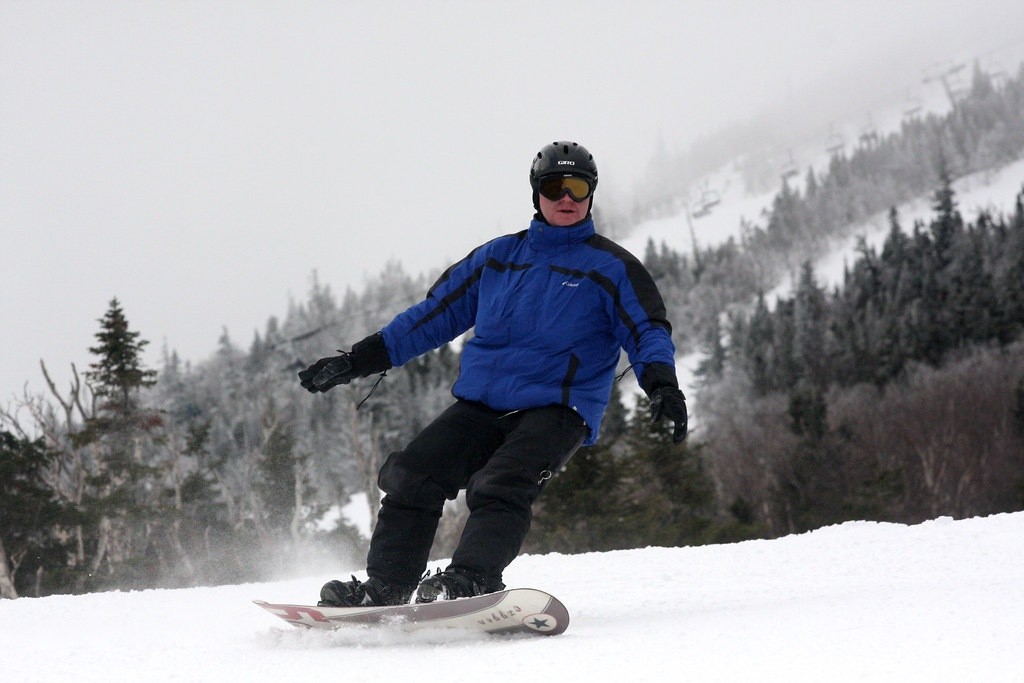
[251,587,569,637]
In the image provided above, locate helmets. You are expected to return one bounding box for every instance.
[530,140,599,191]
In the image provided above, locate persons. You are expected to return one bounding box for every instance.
[298,141,687,606]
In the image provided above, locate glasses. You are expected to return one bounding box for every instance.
[536,173,596,202]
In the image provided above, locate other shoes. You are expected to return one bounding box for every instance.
[416,565,507,604]
[320,575,399,608]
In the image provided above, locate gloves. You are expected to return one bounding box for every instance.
[298,349,363,394]
[648,385,688,445]
